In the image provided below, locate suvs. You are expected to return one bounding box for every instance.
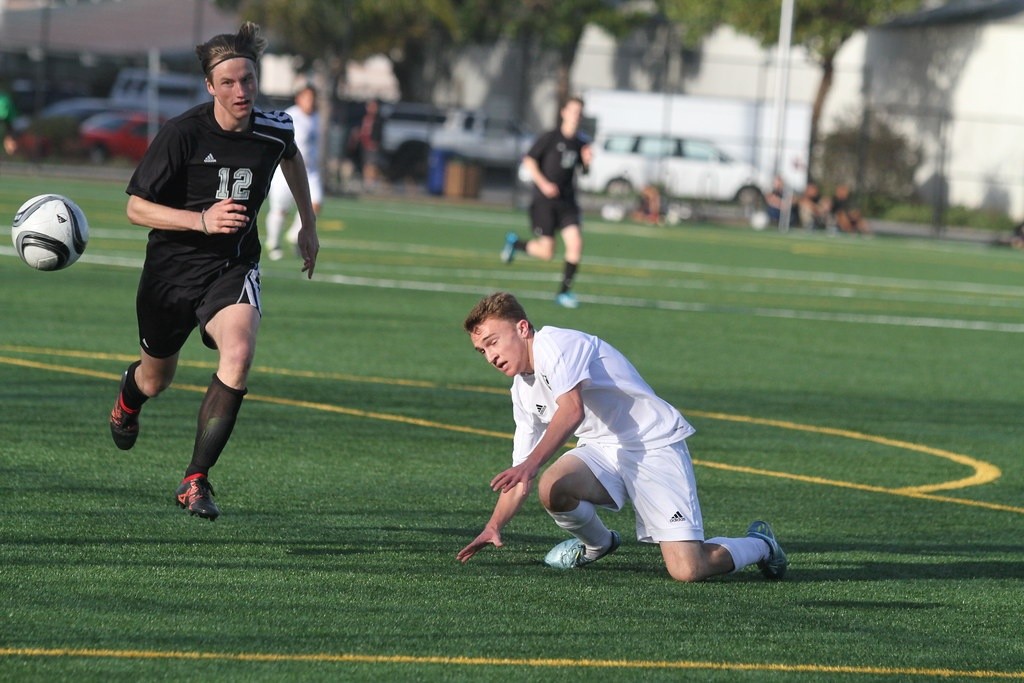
[575,133,779,214]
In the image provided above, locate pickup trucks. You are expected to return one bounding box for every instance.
[378,106,539,185]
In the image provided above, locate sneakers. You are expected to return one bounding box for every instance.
[554,291,578,308]
[545,530,621,568]
[175,477,220,521]
[500,232,519,262]
[745,520,787,580]
[109,370,140,450]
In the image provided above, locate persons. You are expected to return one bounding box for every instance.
[767,178,871,233]
[266,85,323,260]
[109,22,319,518]
[0,78,20,157]
[634,184,670,224]
[360,98,381,197]
[457,293,786,581]
[501,99,591,309]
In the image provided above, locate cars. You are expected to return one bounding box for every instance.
[12,98,171,163]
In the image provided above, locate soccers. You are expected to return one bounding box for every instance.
[12,193,90,271]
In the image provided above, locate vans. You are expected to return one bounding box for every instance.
[110,68,212,119]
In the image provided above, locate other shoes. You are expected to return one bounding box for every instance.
[263,240,282,261]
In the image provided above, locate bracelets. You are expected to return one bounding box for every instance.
[201,210,210,235]
